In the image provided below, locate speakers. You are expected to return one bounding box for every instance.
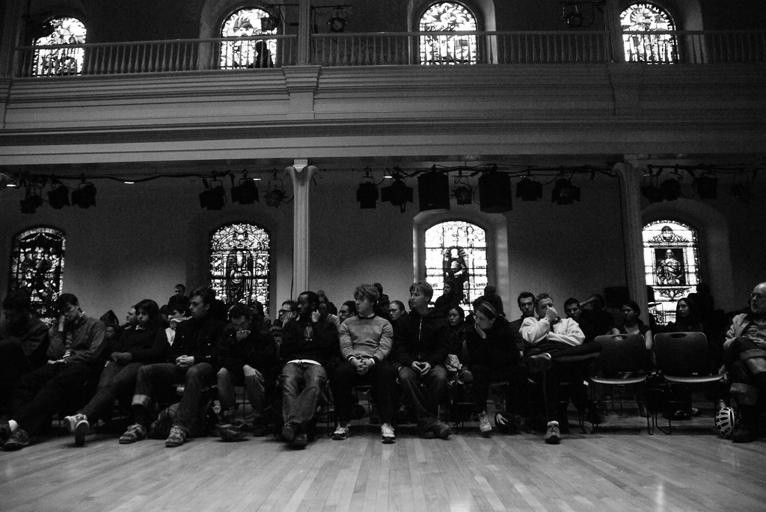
[418,173,450,215]
[479,172,513,213]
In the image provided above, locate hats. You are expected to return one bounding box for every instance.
[478,302,498,319]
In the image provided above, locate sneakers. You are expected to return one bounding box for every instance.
[120,424,147,444]
[478,412,492,433]
[75,420,89,445]
[3,428,29,449]
[64,413,83,432]
[417,425,434,438]
[1,422,10,437]
[165,426,187,446]
[381,423,395,443]
[545,421,560,444]
[733,424,759,442]
[210,420,308,447]
[433,421,449,438]
[332,424,352,440]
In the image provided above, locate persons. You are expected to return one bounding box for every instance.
[249,39,274,68]
[656,247,685,285]
[1,280,764,450]
[227,230,254,301]
[440,246,468,279]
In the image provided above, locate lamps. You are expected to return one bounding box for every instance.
[71,171,99,210]
[638,162,686,204]
[690,166,722,200]
[198,175,228,211]
[231,171,260,206]
[17,178,44,216]
[417,165,450,214]
[477,165,514,214]
[46,174,71,211]
[452,168,474,206]
[550,170,582,206]
[727,168,752,204]
[355,167,379,211]
[515,167,544,201]
[263,167,286,210]
[379,169,416,213]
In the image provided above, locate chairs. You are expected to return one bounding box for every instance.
[2,312,763,442]
